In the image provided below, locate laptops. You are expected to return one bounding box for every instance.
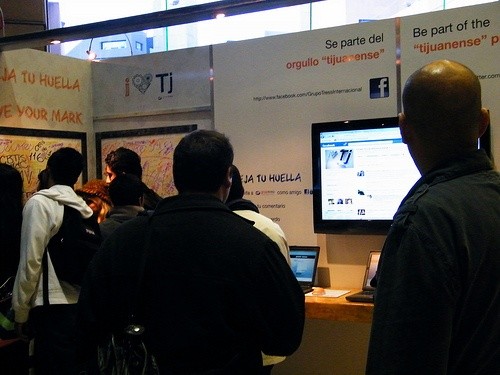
[289,245,321,293]
[345,250,382,303]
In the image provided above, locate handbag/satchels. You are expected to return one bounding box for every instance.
[97,325,153,375]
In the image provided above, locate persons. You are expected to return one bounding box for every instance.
[0,129,305,375]
[366,59,500,375]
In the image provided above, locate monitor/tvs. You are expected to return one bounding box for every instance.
[311,117,490,235]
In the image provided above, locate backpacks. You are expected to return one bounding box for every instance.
[46,204,103,289]
